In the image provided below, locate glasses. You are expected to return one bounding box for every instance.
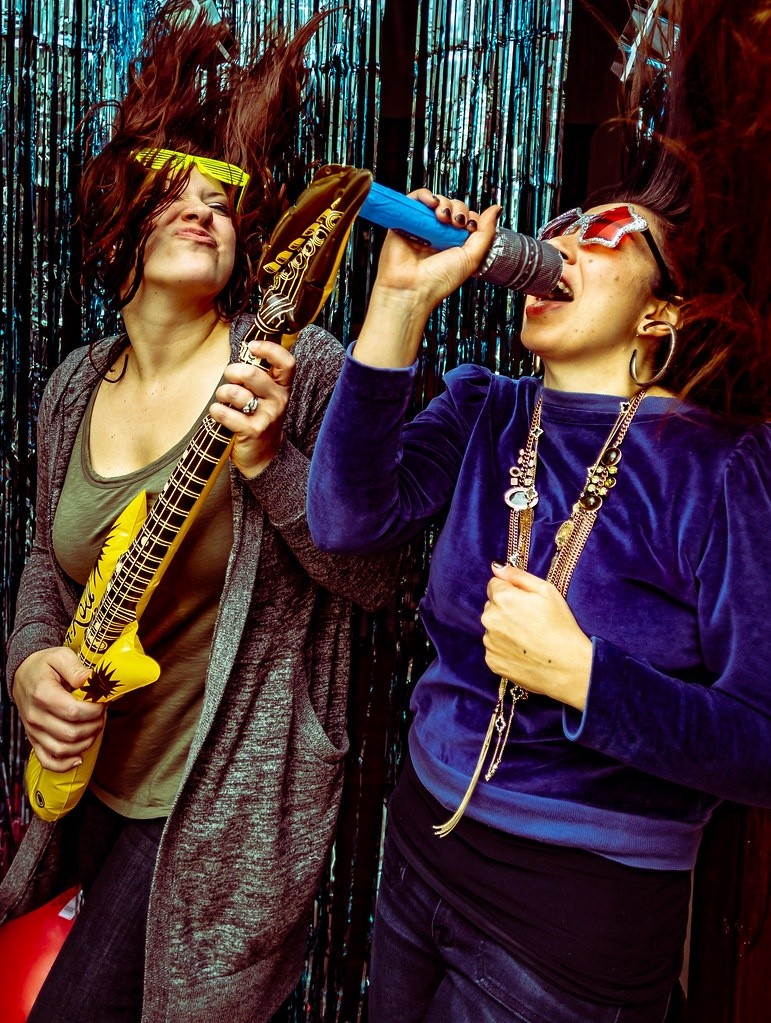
[125,143,251,222]
[533,204,682,302]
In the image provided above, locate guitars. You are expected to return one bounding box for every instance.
[24,167,377,824]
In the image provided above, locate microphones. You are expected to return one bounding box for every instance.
[360,179,561,294]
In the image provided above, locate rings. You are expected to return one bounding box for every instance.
[241,395,260,416]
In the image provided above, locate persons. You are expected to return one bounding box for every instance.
[303,0,771,1023]
[4,2,405,1023]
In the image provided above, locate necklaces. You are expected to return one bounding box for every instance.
[432,382,651,840]
